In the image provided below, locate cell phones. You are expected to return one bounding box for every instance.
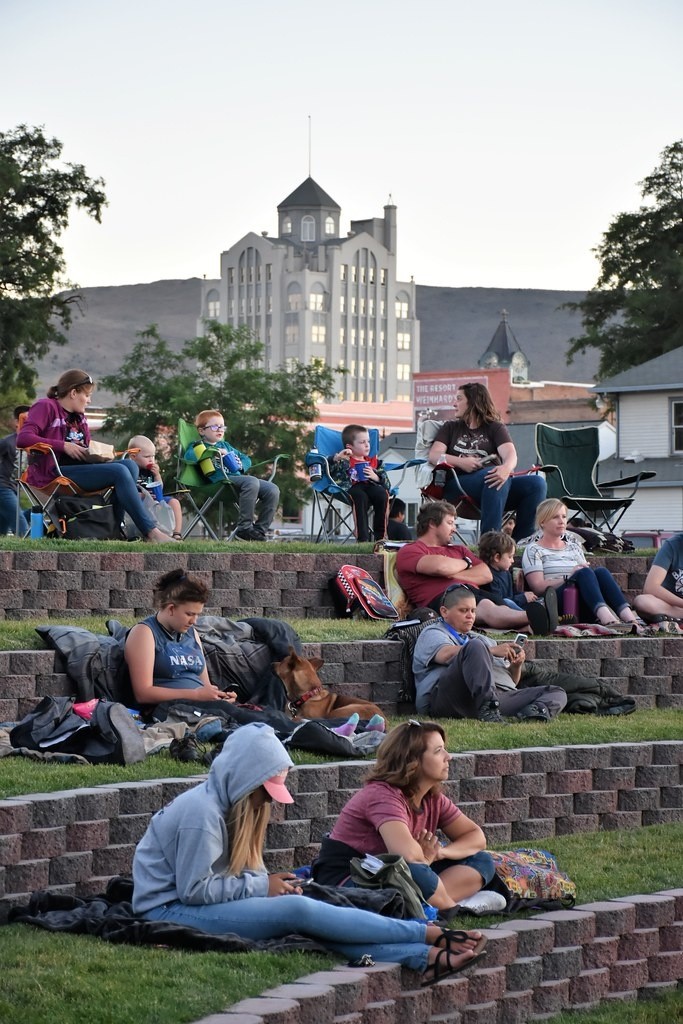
[511,634,527,654]
[218,684,239,700]
[290,878,314,887]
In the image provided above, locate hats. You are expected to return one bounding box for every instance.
[262,766,295,804]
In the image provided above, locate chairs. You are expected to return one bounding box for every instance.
[14,409,291,542]
[306,424,427,546]
[416,420,541,547]
[535,422,656,544]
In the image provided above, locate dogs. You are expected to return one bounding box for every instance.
[269,645,387,734]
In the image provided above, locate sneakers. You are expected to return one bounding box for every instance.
[203,742,223,766]
[455,891,506,914]
[170,734,206,763]
[478,700,512,727]
[514,704,547,724]
[234,527,268,543]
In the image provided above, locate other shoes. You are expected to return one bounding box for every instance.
[545,586,558,633]
[526,601,548,636]
[147,534,180,543]
[601,620,645,632]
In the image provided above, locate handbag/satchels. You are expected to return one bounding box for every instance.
[349,853,433,922]
[55,494,120,541]
[123,484,175,540]
[9,696,146,767]
[484,848,578,913]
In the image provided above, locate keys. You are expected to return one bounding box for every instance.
[358,954,375,966]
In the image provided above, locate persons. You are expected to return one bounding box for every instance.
[326,424,391,542]
[427,382,547,543]
[125,567,387,738]
[185,410,280,542]
[131,722,488,987]
[0,405,31,539]
[129,435,182,541]
[413,583,567,722]
[387,497,411,541]
[634,531,683,620]
[396,498,643,637]
[309,720,507,914]
[16,370,185,544]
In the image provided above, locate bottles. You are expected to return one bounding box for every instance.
[434,454,446,487]
[564,578,577,615]
[513,567,524,594]
[30,505,43,540]
[309,445,322,481]
[193,440,216,476]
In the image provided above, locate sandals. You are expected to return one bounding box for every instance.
[421,947,487,987]
[435,926,487,955]
[173,531,183,542]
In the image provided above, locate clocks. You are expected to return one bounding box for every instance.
[512,354,523,371]
[482,352,499,369]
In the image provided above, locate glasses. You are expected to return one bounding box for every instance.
[203,425,226,432]
[408,718,422,730]
[442,584,469,606]
[70,376,93,388]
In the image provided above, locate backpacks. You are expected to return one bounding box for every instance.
[336,564,400,623]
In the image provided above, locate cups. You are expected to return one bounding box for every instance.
[355,462,369,481]
[146,481,163,502]
[223,451,240,473]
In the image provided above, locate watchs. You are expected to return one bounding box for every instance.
[463,556,473,570]
